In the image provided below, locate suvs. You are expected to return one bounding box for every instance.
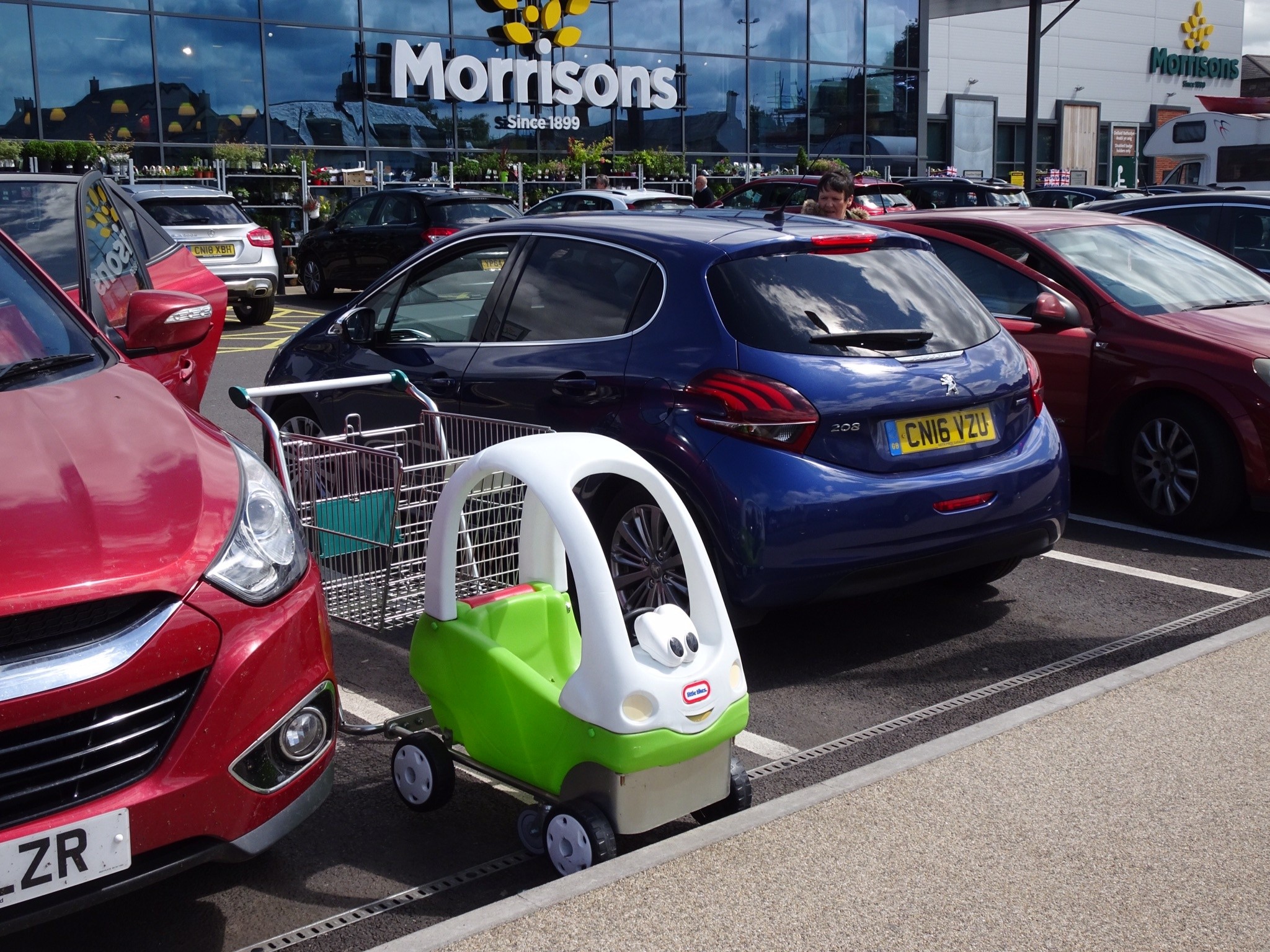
[296,187,525,299]
[894,173,1034,208]
[704,174,917,225]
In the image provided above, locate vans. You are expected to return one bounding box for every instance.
[1133,113,1269,199]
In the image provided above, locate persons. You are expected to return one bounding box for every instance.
[595,174,610,190]
[693,175,715,208]
[800,168,870,220]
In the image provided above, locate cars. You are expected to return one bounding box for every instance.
[260,204,1067,646]
[0,171,341,933]
[845,208,1270,537]
[1027,176,1270,276]
[520,188,699,214]
[117,183,280,325]
[0,173,134,353]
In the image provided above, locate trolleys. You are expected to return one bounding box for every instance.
[228,369,490,751]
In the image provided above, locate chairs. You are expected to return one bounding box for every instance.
[1055,197,1070,208]
[387,200,414,225]
[1234,215,1269,269]
